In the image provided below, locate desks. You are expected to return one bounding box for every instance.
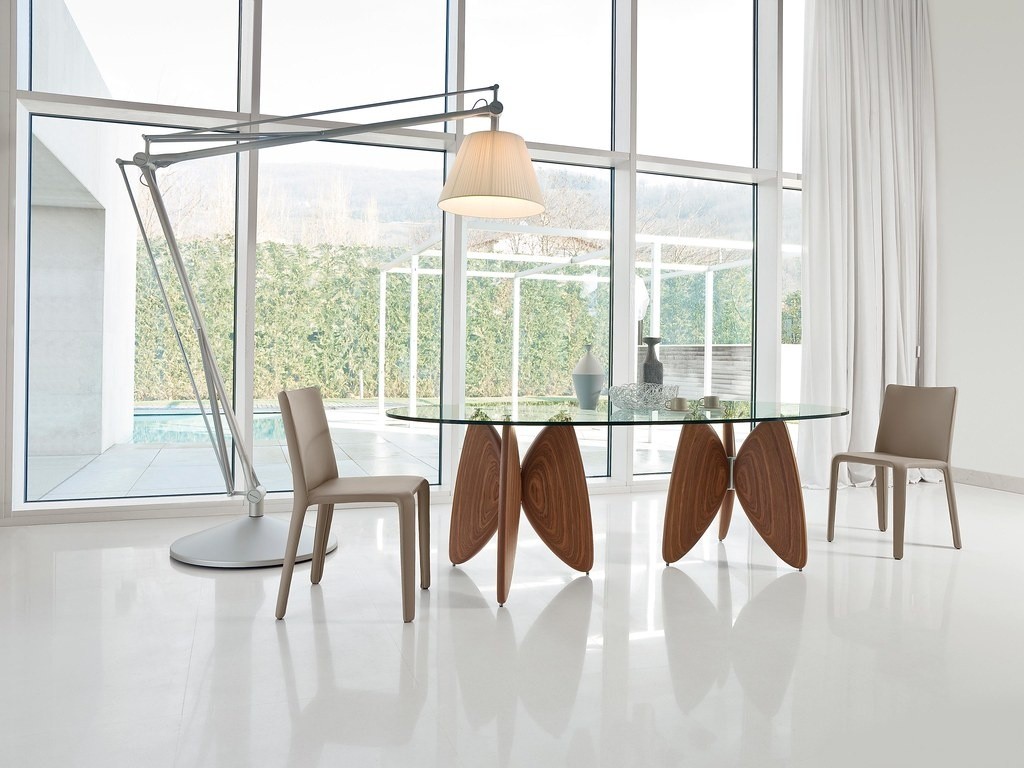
[386,402,851,607]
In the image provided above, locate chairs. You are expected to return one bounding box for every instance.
[276,389,430,623]
[828,384,962,560]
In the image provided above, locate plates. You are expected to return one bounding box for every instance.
[666,409,694,414]
[697,406,726,411]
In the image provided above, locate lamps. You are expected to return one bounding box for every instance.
[117,83,548,569]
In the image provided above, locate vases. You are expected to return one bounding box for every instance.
[635,337,664,400]
[571,345,604,411]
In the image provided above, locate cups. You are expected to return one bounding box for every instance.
[665,398,689,411]
[698,396,720,408]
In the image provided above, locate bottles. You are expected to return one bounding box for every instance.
[639,336,664,384]
[570,344,604,410]
